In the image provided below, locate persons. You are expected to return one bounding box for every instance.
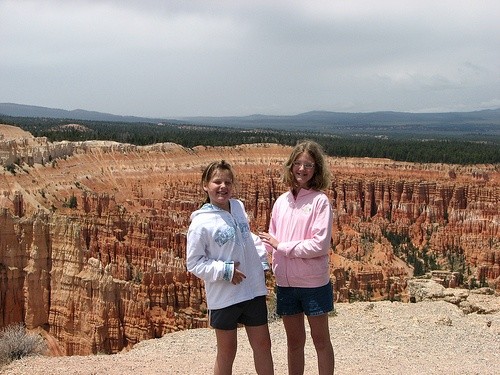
[186,160,274,375]
[258,141,335,375]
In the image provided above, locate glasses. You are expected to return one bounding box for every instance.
[293,161,316,168]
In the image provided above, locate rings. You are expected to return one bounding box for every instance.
[268,238,271,240]
